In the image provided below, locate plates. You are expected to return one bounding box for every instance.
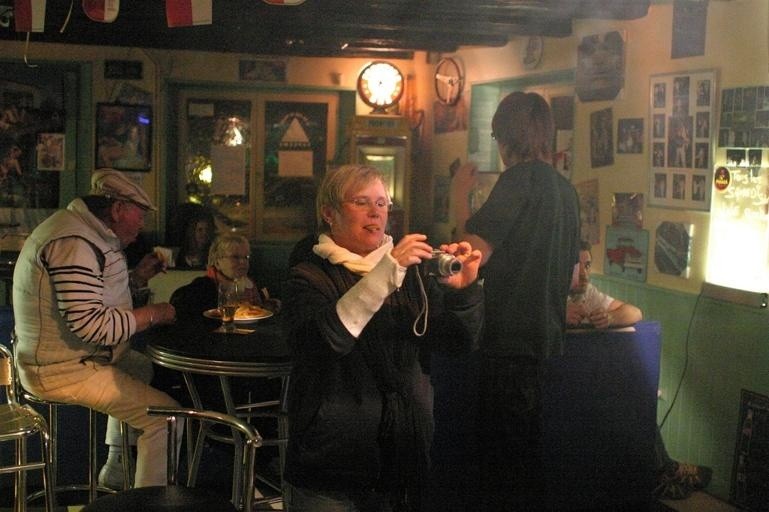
[203,310,274,324]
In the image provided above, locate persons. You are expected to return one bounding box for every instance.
[591,70,769,208]
[450,82,583,384]
[9,171,187,489]
[182,229,283,464]
[176,212,214,269]
[288,168,488,510]
[560,232,716,499]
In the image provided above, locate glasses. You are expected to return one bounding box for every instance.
[215,249,253,264]
[336,197,393,214]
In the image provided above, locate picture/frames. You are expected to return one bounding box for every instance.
[94,100,154,175]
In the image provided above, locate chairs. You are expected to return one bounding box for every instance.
[0,329,264,512]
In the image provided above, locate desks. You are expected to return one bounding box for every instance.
[147,319,299,511]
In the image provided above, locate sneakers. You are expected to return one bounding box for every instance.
[652,455,717,501]
[98,465,128,492]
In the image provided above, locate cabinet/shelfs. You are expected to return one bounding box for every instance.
[169,80,356,281]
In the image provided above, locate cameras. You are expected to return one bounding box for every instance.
[417,249,462,278]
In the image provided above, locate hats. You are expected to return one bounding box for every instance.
[86,166,159,215]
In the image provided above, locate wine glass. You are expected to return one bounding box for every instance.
[217,283,242,332]
